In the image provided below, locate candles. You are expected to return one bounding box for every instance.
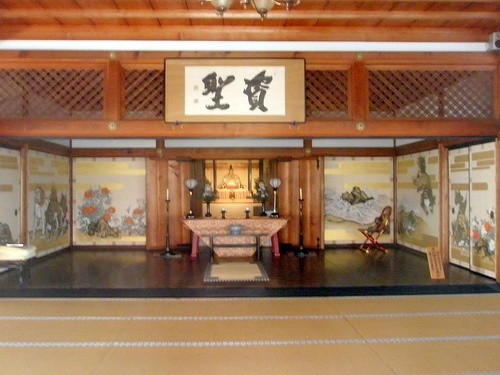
[245,208,250,218]
[222,208,226,219]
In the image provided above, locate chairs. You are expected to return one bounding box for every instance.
[358,206,392,254]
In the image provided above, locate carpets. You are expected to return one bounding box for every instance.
[203,262,270,283]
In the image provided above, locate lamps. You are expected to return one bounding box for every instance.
[269,177,281,217]
[185,178,198,219]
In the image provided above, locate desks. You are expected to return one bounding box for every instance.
[202,231,268,264]
[0,244,37,284]
[181,217,288,258]
